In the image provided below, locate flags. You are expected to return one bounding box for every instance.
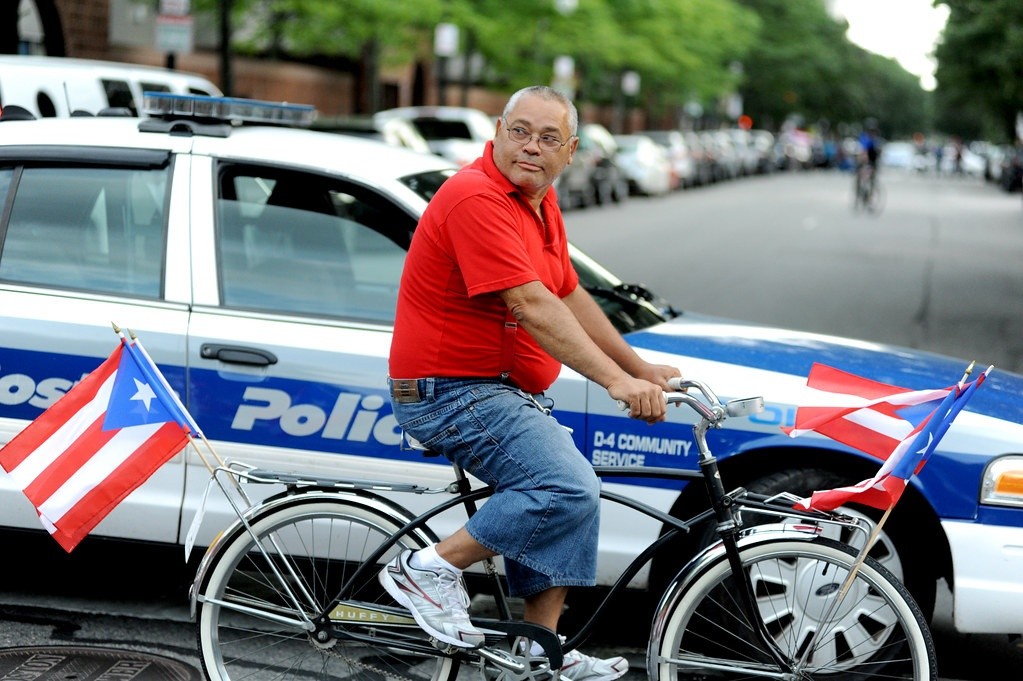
[781,360,986,512]
[0,340,197,552]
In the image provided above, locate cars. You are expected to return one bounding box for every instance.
[235,105,828,264]
[0,91,1023,681]
[0,55,225,255]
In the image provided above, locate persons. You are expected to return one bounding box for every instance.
[379,86,687,681]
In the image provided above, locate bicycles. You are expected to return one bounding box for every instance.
[854,159,882,214]
[185,378,937,681]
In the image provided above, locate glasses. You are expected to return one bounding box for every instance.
[504,117,576,153]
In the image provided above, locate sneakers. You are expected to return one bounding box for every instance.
[378,548,484,648]
[519,634,629,681]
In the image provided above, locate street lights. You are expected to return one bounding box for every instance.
[435,22,458,106]
[620,71,638,135]
